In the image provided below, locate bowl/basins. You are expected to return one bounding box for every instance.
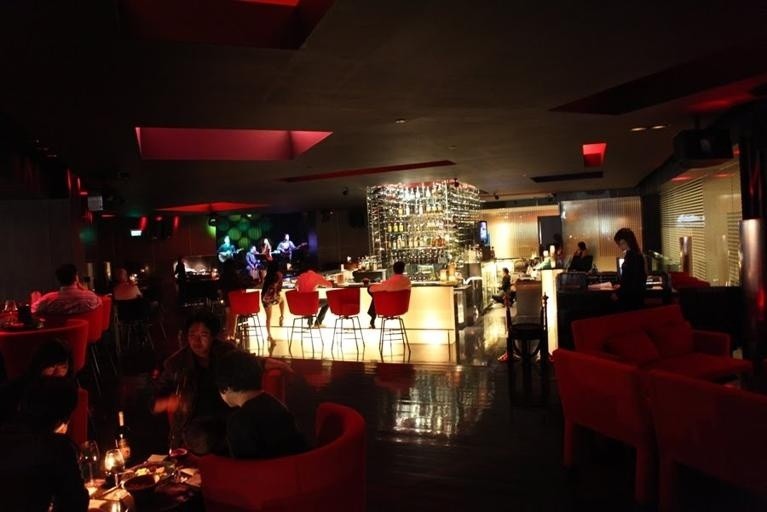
[122,474,160,495]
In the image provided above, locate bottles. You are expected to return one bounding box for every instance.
[117,411,131,463]
[463,244,483,263]
[358,255,378,271]
[367,183,446,264]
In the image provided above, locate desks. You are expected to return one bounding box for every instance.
[557,282,671,317]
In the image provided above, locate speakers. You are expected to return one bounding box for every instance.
[537,215,563,258]
[307,231,319,266]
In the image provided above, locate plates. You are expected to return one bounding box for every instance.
[130,458,172,483]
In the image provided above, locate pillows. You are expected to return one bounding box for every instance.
[604,326,672,371]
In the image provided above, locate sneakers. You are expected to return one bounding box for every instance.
[267,315,283,344]
[309,323,326,328]
[370,319,375,329]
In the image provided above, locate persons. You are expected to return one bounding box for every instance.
[2,233,334,512]
[611,225,647,313]
[490,241,594,307]
[366,261,406,330]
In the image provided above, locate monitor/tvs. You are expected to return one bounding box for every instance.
[478,221,487,243]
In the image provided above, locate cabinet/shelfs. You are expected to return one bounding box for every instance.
[365,178,481,279]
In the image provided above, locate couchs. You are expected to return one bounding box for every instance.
[570,303,752,383]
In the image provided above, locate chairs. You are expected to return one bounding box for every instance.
[285,290,324,359]
[112,286,170,351]
[326,286,365,362]
[98,293,114,332]
[228,288,264,350]
[667,270,711,289]
[640,367,767,511]
[179,272,225,313]
[76,297,109,390]
[553,347,653,500]
[506,278,549,401]
[199,402,369,512]
[0,317,89,388]
[167,355,287,433]
[368,283,411,364]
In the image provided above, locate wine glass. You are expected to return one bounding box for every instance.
[79,438,100,488]
[5,299,18,325]
[104,450,126,499]
[168,434,189,484]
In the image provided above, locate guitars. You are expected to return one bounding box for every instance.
[218,248,244,263]
